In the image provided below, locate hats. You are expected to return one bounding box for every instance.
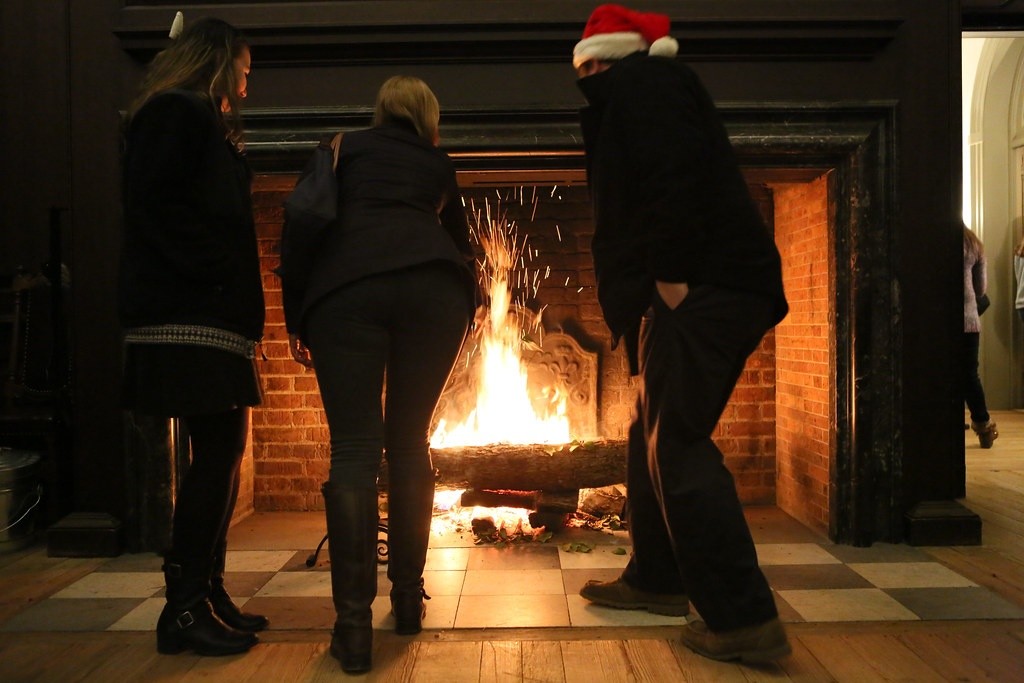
[573,4,679,69]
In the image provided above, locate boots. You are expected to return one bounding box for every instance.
[387,478,435,636]
[320,481,379,671]
[156,557,259,655]
[202,541,269,630]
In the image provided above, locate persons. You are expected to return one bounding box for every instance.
[963,224,998,449]
[1014,238,1024,322]
[272,72,480,676]
[573,4,793,665]
[115,16,271,659]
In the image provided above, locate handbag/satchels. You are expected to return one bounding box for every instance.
[975,290,990,316]
[279,137,339,280]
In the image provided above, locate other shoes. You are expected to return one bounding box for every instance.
[971,421,999,448]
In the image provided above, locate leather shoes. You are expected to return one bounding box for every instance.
[681,616,790,662]
[579,577,690,616]
